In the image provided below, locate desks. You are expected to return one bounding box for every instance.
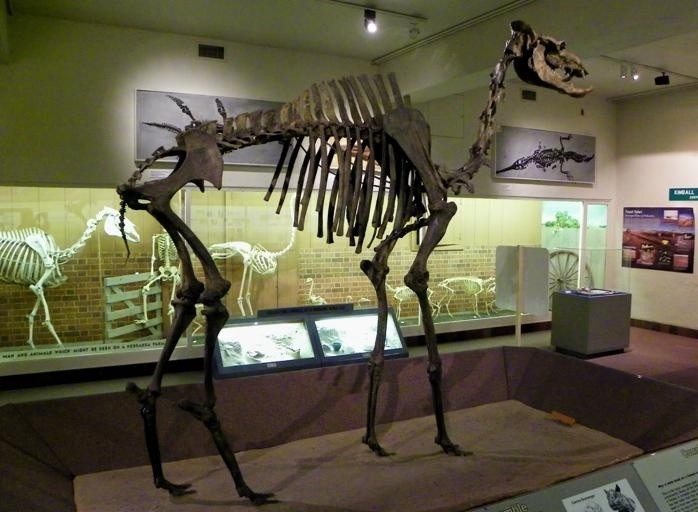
[551,287,631,359]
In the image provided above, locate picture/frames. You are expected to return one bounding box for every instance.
[133,87,296,168]
[494,124,597,185]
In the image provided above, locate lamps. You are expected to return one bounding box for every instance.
[361,8,378,33]
[654,71,670,85]
